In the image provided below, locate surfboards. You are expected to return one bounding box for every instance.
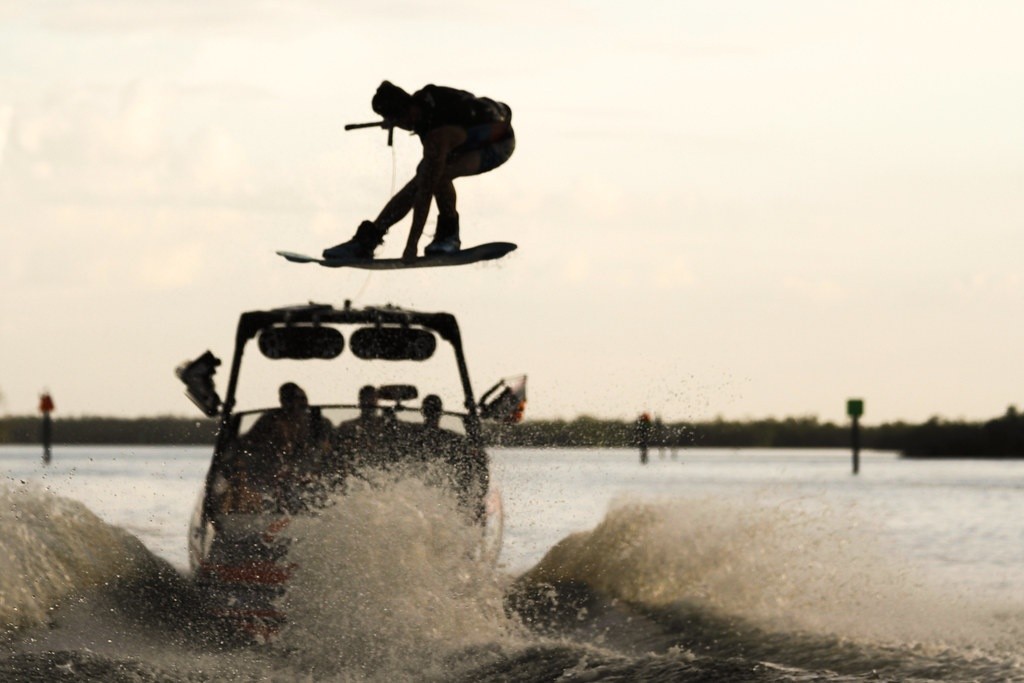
[276,242,517,271]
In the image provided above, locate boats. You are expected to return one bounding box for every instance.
[174,301,529,657]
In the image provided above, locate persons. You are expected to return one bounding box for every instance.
[338,385,399,433]
[321,80,515,265]
[245,380,332,443]
[399,393,464,438]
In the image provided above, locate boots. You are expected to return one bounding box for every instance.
[324,220,387,260]
[425,213,461,254]
